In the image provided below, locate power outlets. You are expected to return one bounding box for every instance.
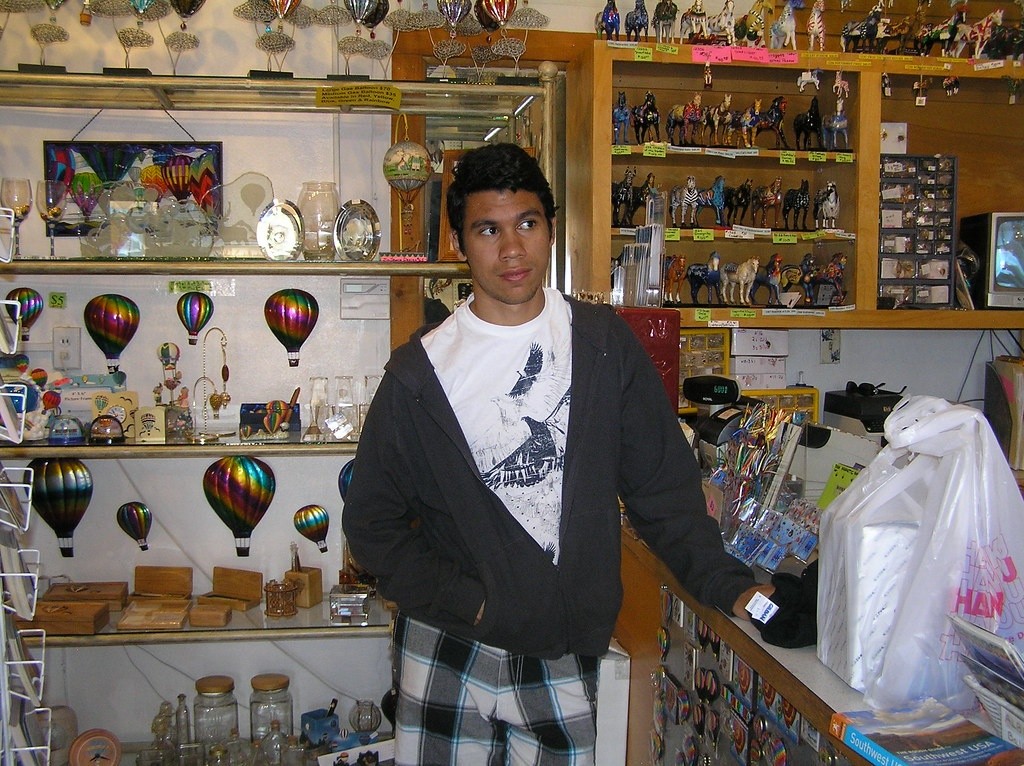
[52,327,81,370]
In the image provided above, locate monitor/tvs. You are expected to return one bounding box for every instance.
[986,212,1024,310]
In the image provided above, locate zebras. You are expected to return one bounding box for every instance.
[670,175,697,226]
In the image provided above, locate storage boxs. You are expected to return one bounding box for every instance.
[12,566,263,635]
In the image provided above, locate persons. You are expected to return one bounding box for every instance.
[342,142,775,766]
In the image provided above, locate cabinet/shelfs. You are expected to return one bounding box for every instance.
[572,39,1024,661]
[0,62,560,766]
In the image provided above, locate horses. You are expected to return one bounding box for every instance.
[667,91,848,152]
[612,92,630,144]
[665,254,687,303]
[595,0,735,45]
[752,176,783,228]
[780,254,819,306]
[720,256,760,305]
[781,178,810,230]
[813,179,841,229]
[750,253,782,305]
[806,0,826,51]
[724,178,753,226]
[686,252,724,304]
[815,253,847,305]
[630,90,660,145]
[611,164,655,226]
[734,0,806,50]
[840,3,1004,61]
[697,175,725,226]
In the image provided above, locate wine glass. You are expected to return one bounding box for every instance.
[1,176,33,258]
[35,180,67,259]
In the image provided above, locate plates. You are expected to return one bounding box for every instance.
[332,199,382,262]
[256,198,305,261]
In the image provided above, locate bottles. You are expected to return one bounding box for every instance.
[299,179,340,263]
[349,698,381,731]
[135,673,294,765]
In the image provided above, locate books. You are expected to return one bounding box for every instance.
[829,697,1024,766]
[944,610,1024,688]
